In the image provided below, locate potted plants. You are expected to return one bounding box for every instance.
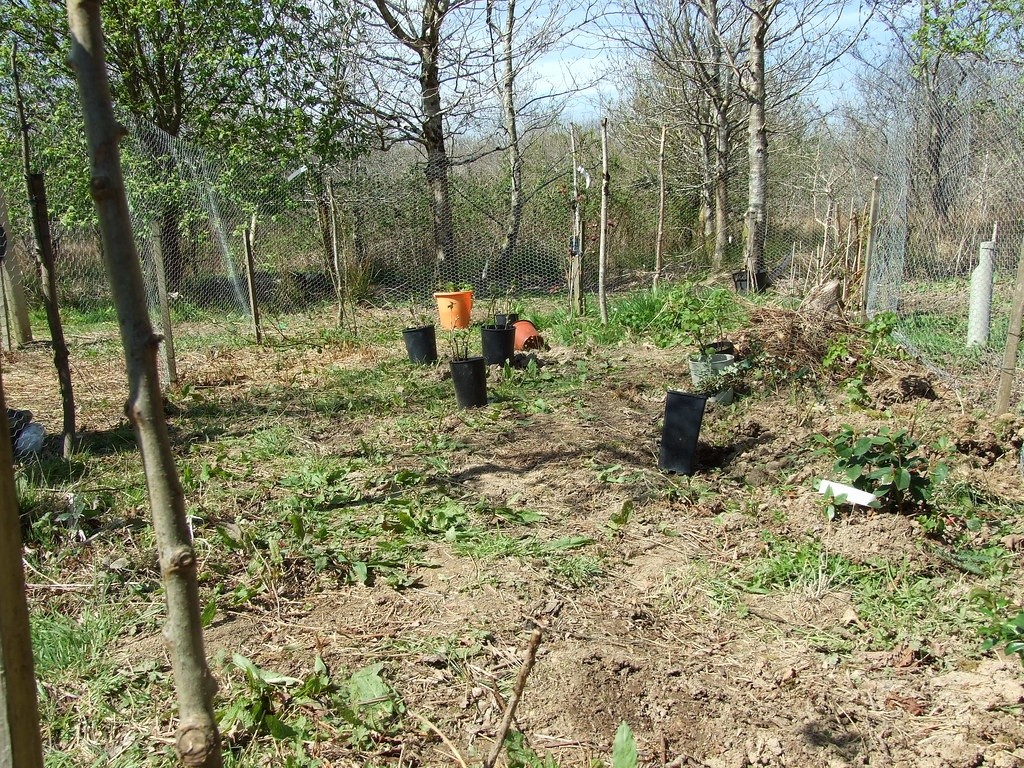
[397,281,742,476]
